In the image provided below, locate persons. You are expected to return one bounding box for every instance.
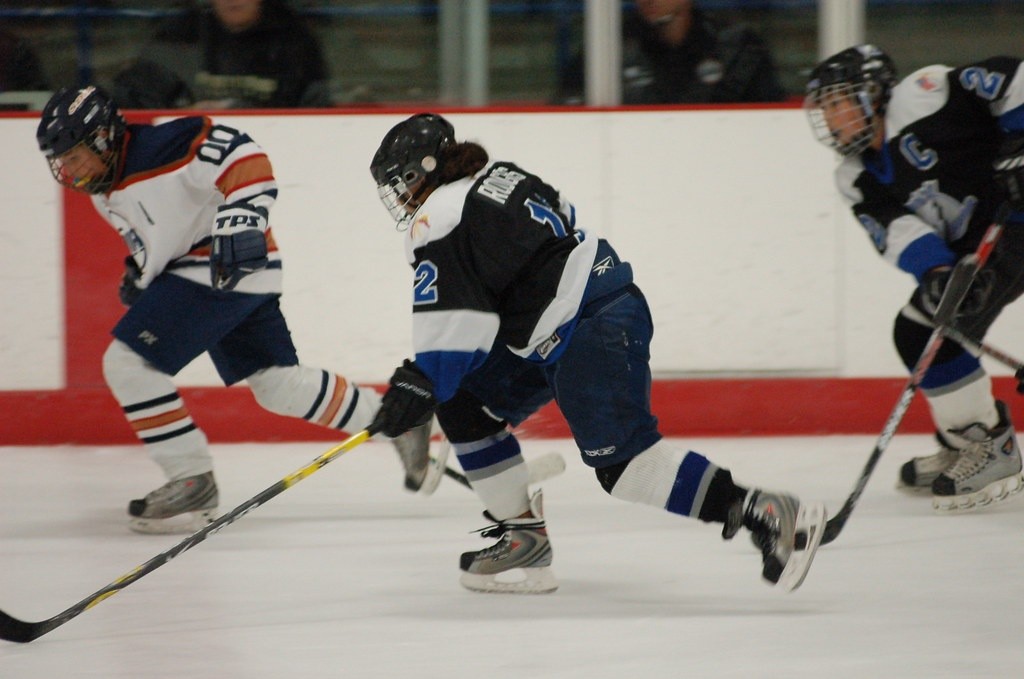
[144,0,333,107]
[804,43,1024,514]
[37,83,452,538]
[370,113,828,597]
[563,0,781,105]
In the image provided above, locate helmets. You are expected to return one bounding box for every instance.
[805,43,897,160]
[370,113,456,230]
[36,84,128,194]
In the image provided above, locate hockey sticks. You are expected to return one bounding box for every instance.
[792,222,1005,552]
[0,415,386,644]
[427,450,567,493]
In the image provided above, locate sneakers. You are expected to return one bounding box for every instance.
[391,410,450,497]
[721,487,829,592]
[128,470,222,533]
[898,431,959,493]
[930,400,1024,510]
[458,489,559,593]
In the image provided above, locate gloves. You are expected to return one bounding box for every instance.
[116,253,144,306]
[988,130,1024,211]
[374,359,437,439]
[209,204,270,292]
[922,258,996,321]
